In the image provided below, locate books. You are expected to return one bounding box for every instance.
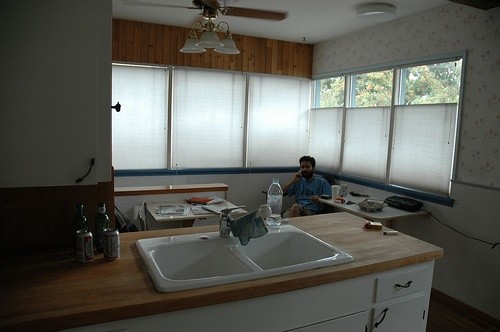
[188,193,224,205]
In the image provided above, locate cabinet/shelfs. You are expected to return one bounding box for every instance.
[69,259,435,332]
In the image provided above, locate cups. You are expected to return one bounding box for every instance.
[331,185,340,200]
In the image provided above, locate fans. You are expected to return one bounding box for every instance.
[126,0,286,21]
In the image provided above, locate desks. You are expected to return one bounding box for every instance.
[146,200,247,227]
[318,194,431,221]
[114,183,228,200]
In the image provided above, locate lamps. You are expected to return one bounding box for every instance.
[180,9,240,54]
[356,3,396,19]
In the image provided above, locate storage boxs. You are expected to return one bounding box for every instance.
[365,221,382,230]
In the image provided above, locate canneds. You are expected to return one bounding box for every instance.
[75,230,94,262]
[341,183,347,196]
[103,228,120,261]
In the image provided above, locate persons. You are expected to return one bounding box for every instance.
[280,155,333,217]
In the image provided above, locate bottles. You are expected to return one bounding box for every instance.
[72,202,90,256]
[266,176,283,228]
[94,202,110,254]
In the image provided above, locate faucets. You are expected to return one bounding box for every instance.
[219,203,273,238]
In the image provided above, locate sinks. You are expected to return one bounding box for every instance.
[135,232,260,294]
[230,224,355,277]
